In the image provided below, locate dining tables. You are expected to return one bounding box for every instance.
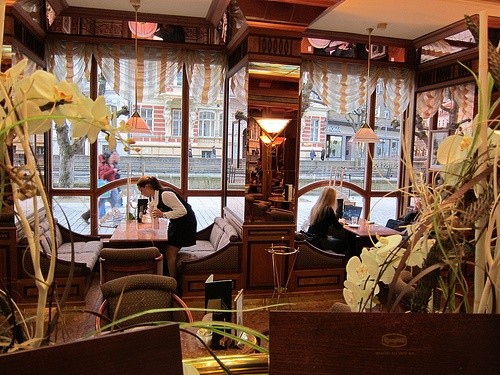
[342,220,403,236]
[180,321,257,359]
[108,218,170,243]
[269,196,294,211]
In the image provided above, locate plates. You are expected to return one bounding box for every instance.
[348,225,360,227]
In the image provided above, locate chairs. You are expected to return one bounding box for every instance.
[99,247,164,284]
[245,191,296,222]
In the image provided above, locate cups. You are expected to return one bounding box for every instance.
[142,215,150,223]
[196,328,213,349]
[351,216,358,227]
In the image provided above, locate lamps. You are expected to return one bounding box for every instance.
[253,118,292,141]
[348,28,382,143]
[258,136,286,154]
[119,1,154,134]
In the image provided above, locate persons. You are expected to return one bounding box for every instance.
[321,147,326,161]
[137,175,198,281]
[305,185,361,259]
[385,198,423,233]
[188,144,193,158]
[255,158,263,179]
[81,150,123,225]
[271,158,289,186]
[310,149,316,160]
[212,146,216,158]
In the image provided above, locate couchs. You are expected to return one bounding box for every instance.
[96,274,193,334]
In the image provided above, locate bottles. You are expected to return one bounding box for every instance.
[152,206,160,230]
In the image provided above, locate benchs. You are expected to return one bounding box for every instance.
[293,241,346,290]
[17,217,104,301]
[176,207,244,297]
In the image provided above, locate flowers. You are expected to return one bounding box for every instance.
[0,58,297,375]
[344,12,500,315]
